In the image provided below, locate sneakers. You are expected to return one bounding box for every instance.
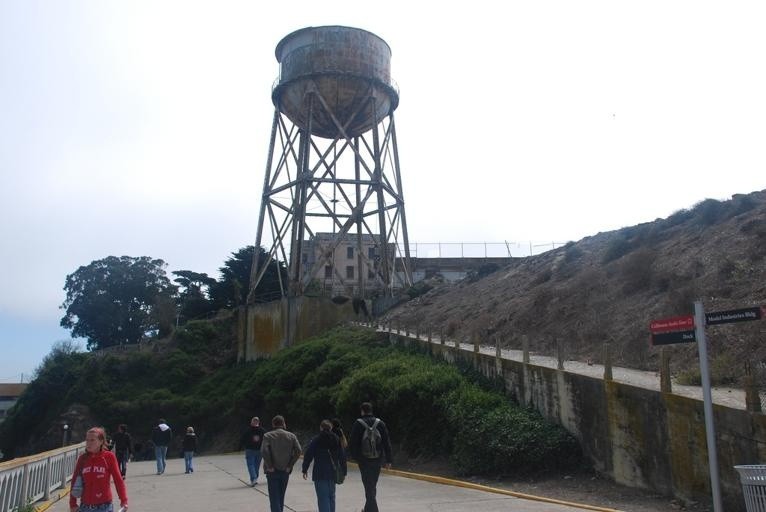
[246,479,258,487]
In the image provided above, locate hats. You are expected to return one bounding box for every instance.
[272,415,285,426]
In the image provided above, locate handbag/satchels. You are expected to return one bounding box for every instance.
[71,471,83,498]
[334,464,345,484]
[341,435,348,449]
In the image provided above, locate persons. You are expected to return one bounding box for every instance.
[302,419,348,512]
[69,426,128,511]
[150,419,172,475]
[239,416,267,488]
[180,426,197,474]
[349,401,392,512]
[259,415,302,512]
[331,418,347,476]
[100,423,134,481]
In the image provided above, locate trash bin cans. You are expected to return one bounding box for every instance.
[733,463,766,512]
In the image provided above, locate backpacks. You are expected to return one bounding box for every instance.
[356,418,386,459]
